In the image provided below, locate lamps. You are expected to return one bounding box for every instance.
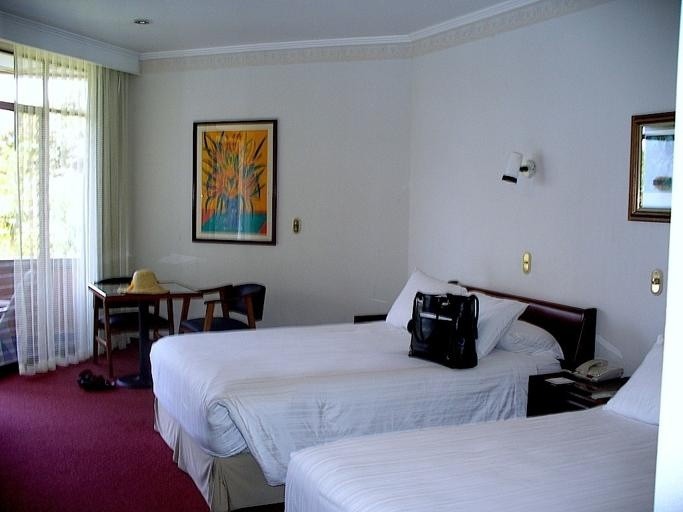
[502,152,536,185]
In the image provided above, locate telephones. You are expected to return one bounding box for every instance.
[575,359,624,382]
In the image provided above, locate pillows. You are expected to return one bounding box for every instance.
[386,267,468,328]
[496,321,565,361]
[602,334,664,426]
[465,291,529,360]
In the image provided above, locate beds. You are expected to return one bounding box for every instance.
[284,334,664,511]
[150,281,597,511]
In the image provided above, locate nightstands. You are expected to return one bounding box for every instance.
[354,314,388,323]
[527,371,631,417]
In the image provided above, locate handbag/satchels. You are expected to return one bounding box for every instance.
[407,292,479,368]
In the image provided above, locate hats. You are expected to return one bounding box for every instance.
[117,271,169,294]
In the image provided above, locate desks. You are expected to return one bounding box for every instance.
[88,282,202,389]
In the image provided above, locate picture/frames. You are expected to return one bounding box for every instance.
[628,111,675,223]
[192,120,277,246]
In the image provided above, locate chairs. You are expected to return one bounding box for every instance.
[93,277,174,378]
[178,284,266,334]
[0,270,36,356]
